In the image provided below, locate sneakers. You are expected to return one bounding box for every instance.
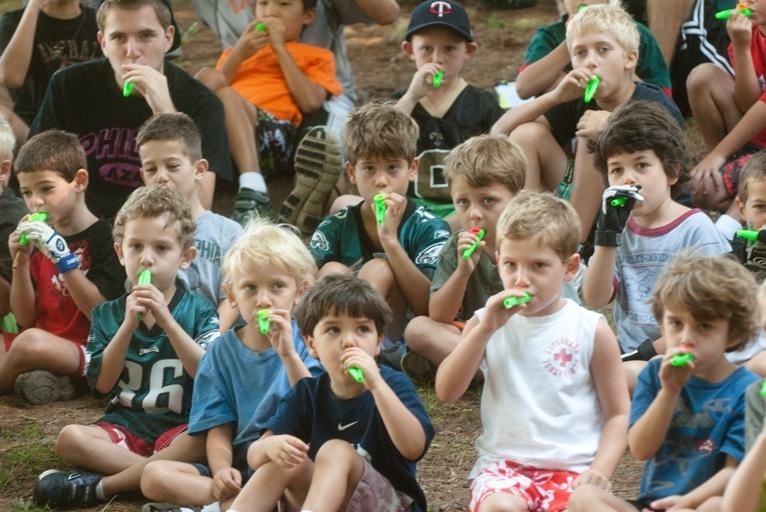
[279,125,342,235]
[141,504,181,512]
[16,371,75,405]
[34,470,102,508]
[400,352,437,381]
[231,187,272,226]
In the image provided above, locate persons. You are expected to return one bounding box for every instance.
[434,189,631,512]
[312,2,763,405]
[136,216,326,507]
[34,186,222,508]
[0,1,400,403]
[720,377,766,512]
[564,251,763,512]
[218,274,433,512]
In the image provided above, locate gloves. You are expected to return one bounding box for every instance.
[20,221,79,274]
[732,229,765,283]
[594,184,645,246]
[622,340,657,362]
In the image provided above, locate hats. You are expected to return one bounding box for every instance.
[405,0,474,42]
